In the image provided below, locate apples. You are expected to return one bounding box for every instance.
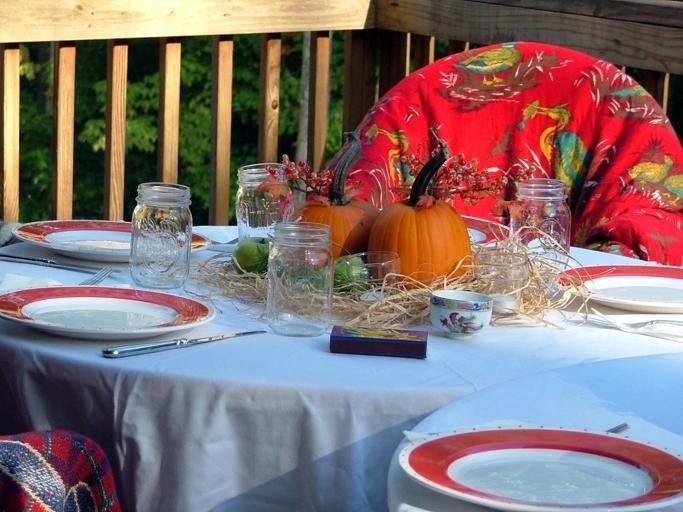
[231,236,271,274]
[329,254,369,292]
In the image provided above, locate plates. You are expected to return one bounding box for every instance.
[12,220,210,264]
[0,285,216,342]
[557,265,683,316]
[460,214,512,255]
[398,425,681,512]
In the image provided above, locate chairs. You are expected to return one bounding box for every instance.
[306,39,681,262]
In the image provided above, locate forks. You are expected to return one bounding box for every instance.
[75,265,115,286]
[590,305,682,332]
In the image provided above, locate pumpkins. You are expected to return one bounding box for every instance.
[367,129,474,294]
[288,131,380,270]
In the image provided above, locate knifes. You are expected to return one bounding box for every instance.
[1,252,123,278]
[99,328,268,358]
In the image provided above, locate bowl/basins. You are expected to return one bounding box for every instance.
[429,290,493,341]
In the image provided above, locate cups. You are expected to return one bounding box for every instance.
[347,251,401,303]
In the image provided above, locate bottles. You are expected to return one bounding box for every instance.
[130,182,194,291]
[510,178,571,269]
[268,221,335,337]
[235,162,295,242]
